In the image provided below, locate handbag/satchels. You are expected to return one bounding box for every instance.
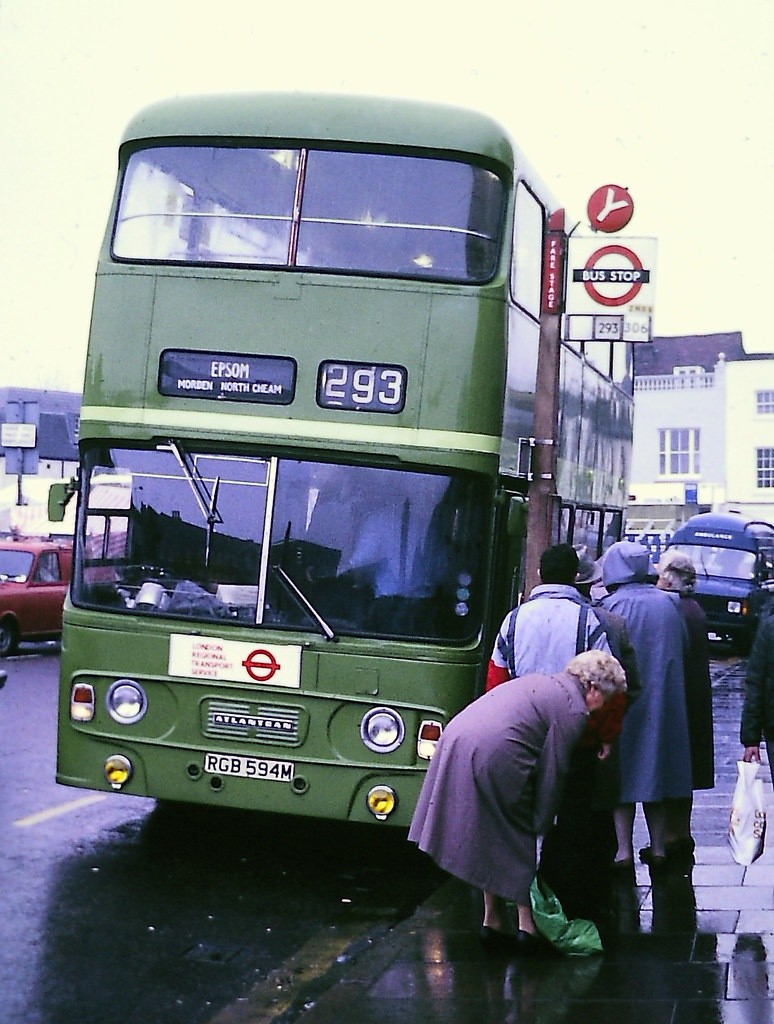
[728,759,768,865]
[530,873,603,958]
[167,580,231,618]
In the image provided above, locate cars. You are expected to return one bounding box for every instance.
[0,540,116,662]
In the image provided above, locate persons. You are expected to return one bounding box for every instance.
[572,544,642,709]
[638,550,714,861]
[486,544,620,913]
[739,614,774,791]
[597,541,693,871]
[407,649,627,951]
[347,512,447,598]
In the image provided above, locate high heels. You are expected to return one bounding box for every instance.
[515,930,553,952]
[481,926,515,945]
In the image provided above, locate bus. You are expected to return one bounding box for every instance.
[47,89,638,840]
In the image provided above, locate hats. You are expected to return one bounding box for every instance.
[573,544,604,584]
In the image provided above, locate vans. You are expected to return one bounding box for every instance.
[653,509,774,654]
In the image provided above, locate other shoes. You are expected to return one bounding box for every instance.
[639,847,669,868]
[613,858,633,869]
